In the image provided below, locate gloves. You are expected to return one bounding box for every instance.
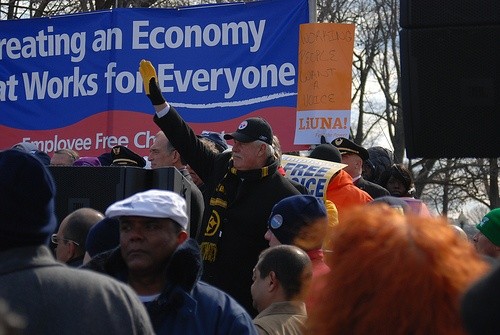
[139,60,165,105]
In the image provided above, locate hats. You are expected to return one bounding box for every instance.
[331,137,369,161]
[85,217,119,255]
[307,144,342,163]
[106,189,186,230]
[1,149,58,234]
[196,131,228,150]
[110,146,146,167]
[268,195,327,250]
[476,208,499,246]
[224,116,273,145]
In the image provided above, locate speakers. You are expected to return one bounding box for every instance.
[399,0,500,159]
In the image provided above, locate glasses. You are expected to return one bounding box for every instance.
[51,234,80,247]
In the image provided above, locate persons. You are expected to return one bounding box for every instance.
[138,59,304,320]
[0,131,500,335]
